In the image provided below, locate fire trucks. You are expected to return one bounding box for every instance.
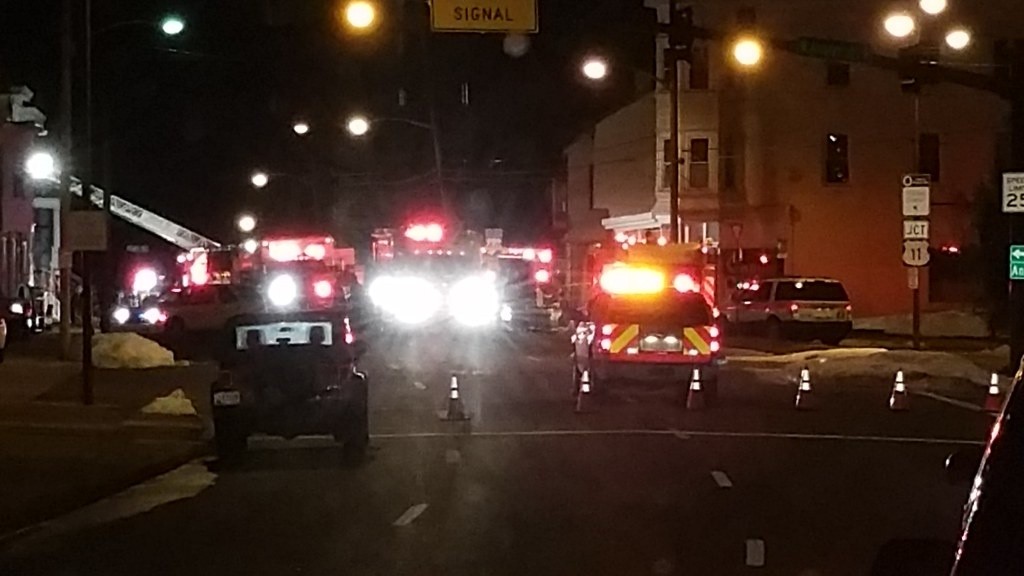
[51,168,365,318]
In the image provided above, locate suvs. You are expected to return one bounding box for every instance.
[727,277,853,347]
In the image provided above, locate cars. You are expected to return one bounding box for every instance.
[101,297,181,346]
[238,257,384,356]
[154,283,263,330]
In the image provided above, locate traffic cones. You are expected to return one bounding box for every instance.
[793,365,819,407]
[439,374,472,421]
[886,370,909,411]
[980,370,1004,411]
[574,369,593,413]
[683,363,707,412]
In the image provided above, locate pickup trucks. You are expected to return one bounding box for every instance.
[207,310,370,467]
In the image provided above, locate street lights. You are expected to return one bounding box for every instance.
[250,167,321,235]
[883,0,970,348]
[583,59,680,244]
[23,151,95,403]
[61,11,186,147]
[350,118,458,239]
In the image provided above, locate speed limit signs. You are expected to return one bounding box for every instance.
[1003,172,1024,212]
